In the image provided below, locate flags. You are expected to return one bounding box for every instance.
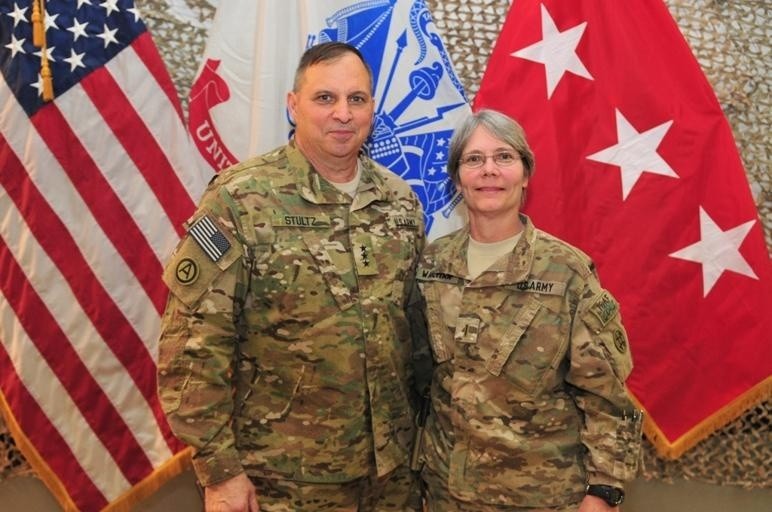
[469,0,772,465]
[187,0,476,244]
[1,0,208,511]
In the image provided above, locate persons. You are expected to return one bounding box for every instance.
[410,109,645,512]
[155,41,427,511]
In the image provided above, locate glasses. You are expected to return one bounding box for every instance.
[458,151,524,169]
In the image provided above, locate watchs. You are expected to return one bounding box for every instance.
[585,483,626,507]
